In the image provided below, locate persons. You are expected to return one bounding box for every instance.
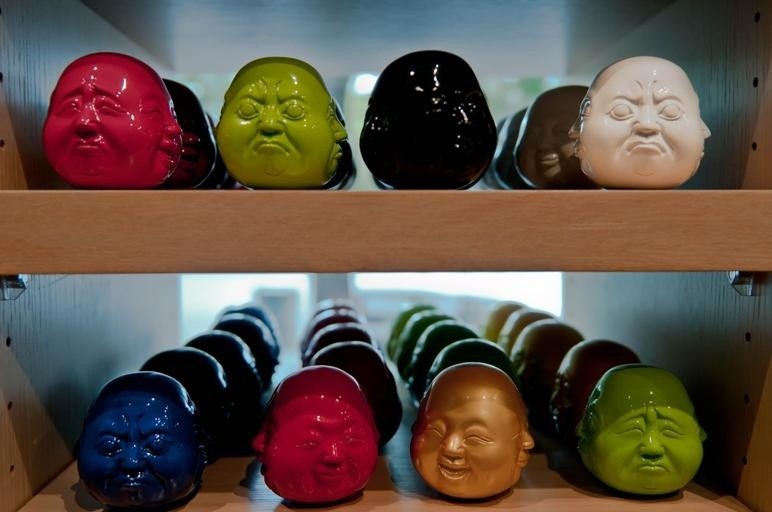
[43,51,712,190]
[70,298,708,510]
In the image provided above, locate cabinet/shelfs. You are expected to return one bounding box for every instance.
[0,0,771,512]
